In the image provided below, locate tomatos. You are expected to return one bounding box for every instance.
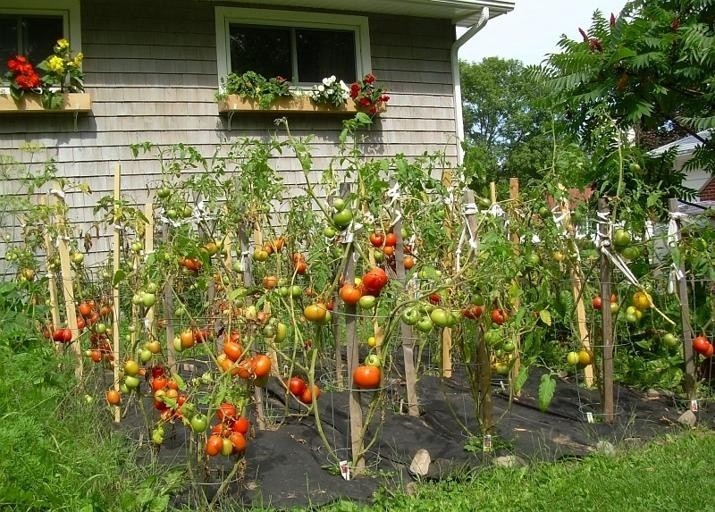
[5,161,715,458]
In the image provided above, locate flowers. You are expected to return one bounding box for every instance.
[222,70,292,108]
[4,54,42,101]
[347,74,391,113]
[309,75,350,109]
[38,37,88,109]
